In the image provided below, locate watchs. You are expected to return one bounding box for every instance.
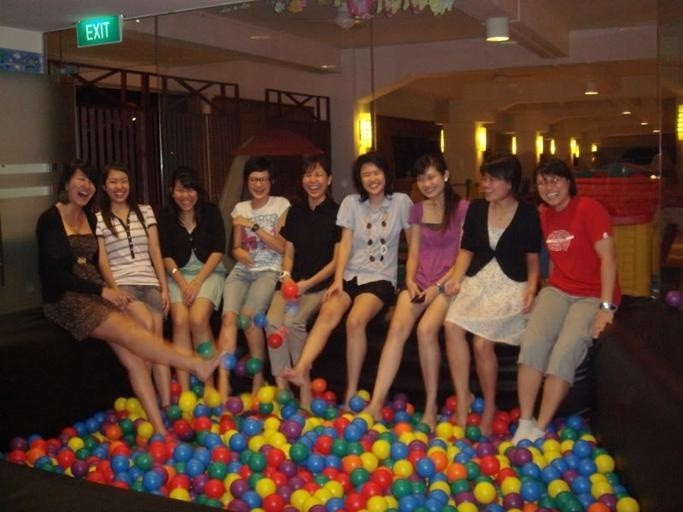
[436,282,444,292]
[250,223,259,232]
[279,272,289,280]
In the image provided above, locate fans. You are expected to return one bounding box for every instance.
[289,0,366,30]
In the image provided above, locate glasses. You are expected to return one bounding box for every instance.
[248,176,269,184]
[536,178,562,187]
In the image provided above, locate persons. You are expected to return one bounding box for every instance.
[94,162,172,409]
[36,158,227,436]
[363,149,472,434]
[219,156,291,396]
[279,153,415,413]
[443,154,542,439]
[265,153,340,411]
[511,157,624,448]
[157,169,228,392]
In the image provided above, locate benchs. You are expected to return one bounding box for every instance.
[1,263,597,453]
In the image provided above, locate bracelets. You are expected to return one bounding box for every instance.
[170,268,178,274]
[598,303,619,312]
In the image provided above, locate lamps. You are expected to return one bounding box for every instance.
[478,0,523,44]
